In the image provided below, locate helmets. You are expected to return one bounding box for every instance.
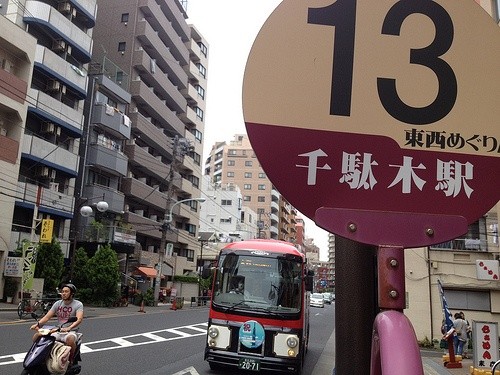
[63,284,76,294]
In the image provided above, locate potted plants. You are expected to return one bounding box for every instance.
[5,279,21,303]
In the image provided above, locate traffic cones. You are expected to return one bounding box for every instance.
[169,296,178,310]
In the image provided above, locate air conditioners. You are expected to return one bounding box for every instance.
[51,40,72,57]
[46,79,67,95]
[58,2,76,18]
[39,167,57,180]
[41,122,61,137]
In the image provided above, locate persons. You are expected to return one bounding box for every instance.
[30,283,83,367]
[230,279,250,297]
[441,311,471,359]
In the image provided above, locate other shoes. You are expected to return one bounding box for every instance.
[464,355,470,359]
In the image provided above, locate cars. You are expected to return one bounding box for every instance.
[309,293,335,308]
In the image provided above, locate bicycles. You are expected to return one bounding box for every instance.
[18,287,61,318]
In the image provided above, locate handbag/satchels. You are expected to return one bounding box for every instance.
[440,339,448,349]
[455,328,461,336]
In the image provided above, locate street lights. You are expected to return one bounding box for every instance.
[153,197,206,307]
[69,193,109,282]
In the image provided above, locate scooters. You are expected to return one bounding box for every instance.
[19,312,83,375]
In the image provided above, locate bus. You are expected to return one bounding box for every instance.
[202,239,313,375]
